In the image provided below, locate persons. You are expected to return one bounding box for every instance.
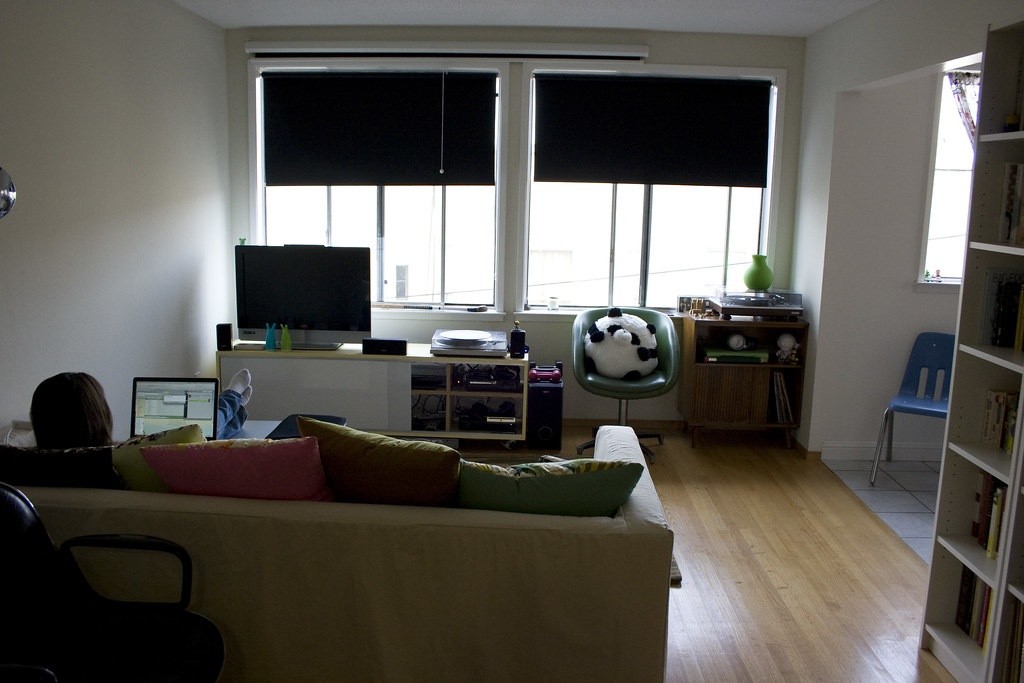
[776,333,801,366]
[28,367,253,447]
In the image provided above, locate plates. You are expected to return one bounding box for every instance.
[440,330,491,340]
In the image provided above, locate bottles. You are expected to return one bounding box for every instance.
[744,255,774,290]
[925,270,941,282]
[548,297,559,310]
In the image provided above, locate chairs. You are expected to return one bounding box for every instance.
[869,332,955,483]
[0,481,226,683]
[572,308,680,465]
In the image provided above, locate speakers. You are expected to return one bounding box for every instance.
[528,380,564,451]
[216,324,234,351]
[362,338,407,355]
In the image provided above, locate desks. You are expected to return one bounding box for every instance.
[216,342,529,447]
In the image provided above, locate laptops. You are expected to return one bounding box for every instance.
[130,377,219,441]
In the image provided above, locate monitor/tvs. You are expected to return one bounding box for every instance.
[235,243,371,351]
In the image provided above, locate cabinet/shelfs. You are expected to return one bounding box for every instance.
[920,19,1024,683]
[677,315,810,448]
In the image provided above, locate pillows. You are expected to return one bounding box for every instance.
[0,403,644,519]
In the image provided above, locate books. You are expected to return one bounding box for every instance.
[997,600,1024,683]
[971,470,1008,561]
[773,371,794,425]
[999,159,1024,246]
[955,564,995,657]
[981,387,1020,457]
[976,268,1024,354]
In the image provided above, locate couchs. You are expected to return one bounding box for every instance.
[0,425,674,683]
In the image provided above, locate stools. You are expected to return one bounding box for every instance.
[225,420,282,439]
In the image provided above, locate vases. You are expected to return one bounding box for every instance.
[746,255,773,290]
